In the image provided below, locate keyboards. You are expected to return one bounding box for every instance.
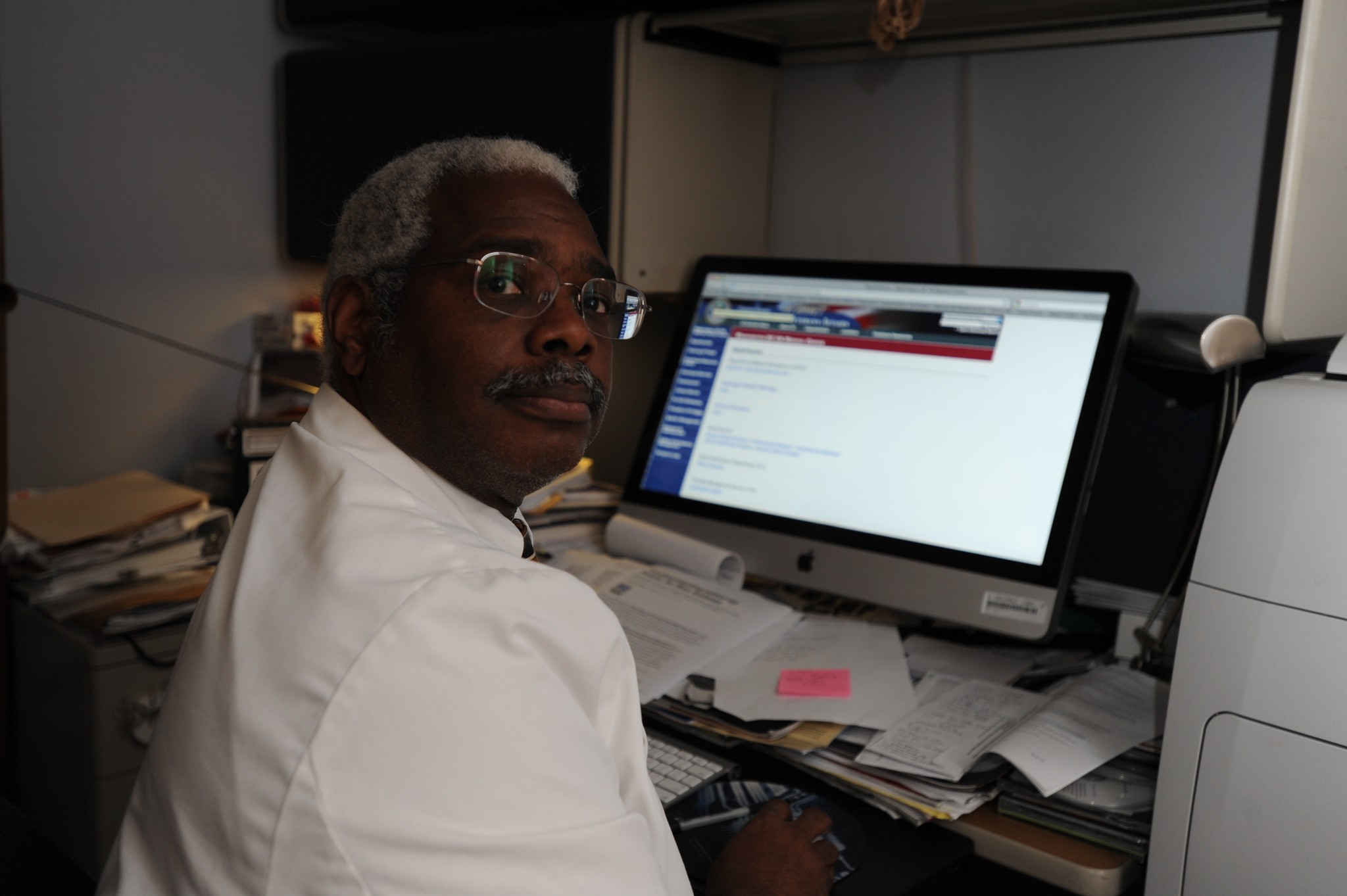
[643,727,739,814]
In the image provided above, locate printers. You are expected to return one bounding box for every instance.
[1146,371,1346,893]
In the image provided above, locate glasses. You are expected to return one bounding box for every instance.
[411,251,653,341]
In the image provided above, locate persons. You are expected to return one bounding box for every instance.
[111,135,844,895]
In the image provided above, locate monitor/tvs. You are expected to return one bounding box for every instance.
[616,254,1139,643]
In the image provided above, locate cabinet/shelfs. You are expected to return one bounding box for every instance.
[604,3,780,299]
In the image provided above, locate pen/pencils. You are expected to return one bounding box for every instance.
[670,801,770,834]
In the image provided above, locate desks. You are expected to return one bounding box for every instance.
[525,484,1157,892]
